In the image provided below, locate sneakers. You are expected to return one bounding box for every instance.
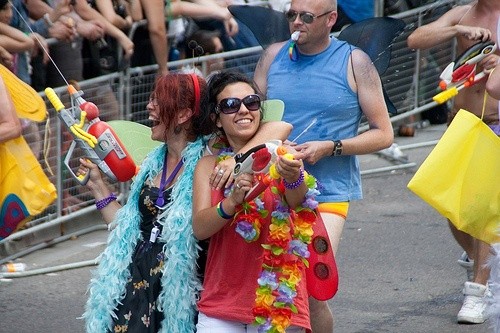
[458,250,475,283]
[458,281,495,323]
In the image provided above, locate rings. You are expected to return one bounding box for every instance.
[218,170,224,176]
[215,167,219,170]
[236,184,240,189]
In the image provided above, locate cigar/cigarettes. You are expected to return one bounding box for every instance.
[230,36,235,44]
[101,38,107,45]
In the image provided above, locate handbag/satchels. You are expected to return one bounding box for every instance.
[407,67,500,243]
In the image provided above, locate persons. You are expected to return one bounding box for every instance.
[253,1,394,333]
[407,0,500,324]
[192,72,320,333]
[76,73,293,333]
[0,0,409,252]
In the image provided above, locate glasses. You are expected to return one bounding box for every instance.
[286,9,332,23]
[215,95,261,114]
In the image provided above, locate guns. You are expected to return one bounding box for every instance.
[432,40,498,105]
[224,139,293,202]
[44,84,136,186]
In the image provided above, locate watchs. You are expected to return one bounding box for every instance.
[332,139,343,157]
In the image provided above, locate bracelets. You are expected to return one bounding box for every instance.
[96,194,117,209]
[44,14,52,25]
[283,168,304,189]
[217,201,234,219]
